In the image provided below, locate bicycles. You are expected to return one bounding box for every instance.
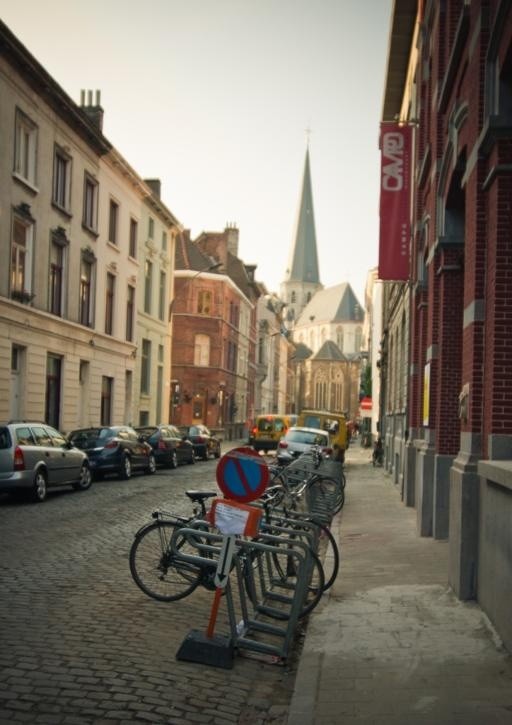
[128,446,346,622]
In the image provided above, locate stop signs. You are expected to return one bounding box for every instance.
[360,395,373,411]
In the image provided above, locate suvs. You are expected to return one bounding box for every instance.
[296,409,350,465]
[0,419,94,501]
[132,424,196,470]
[63,425,158,480]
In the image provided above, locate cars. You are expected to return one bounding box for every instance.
[177,424,222,462]
[274,426,332,467]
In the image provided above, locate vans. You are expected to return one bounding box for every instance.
[252,414,294,453]
